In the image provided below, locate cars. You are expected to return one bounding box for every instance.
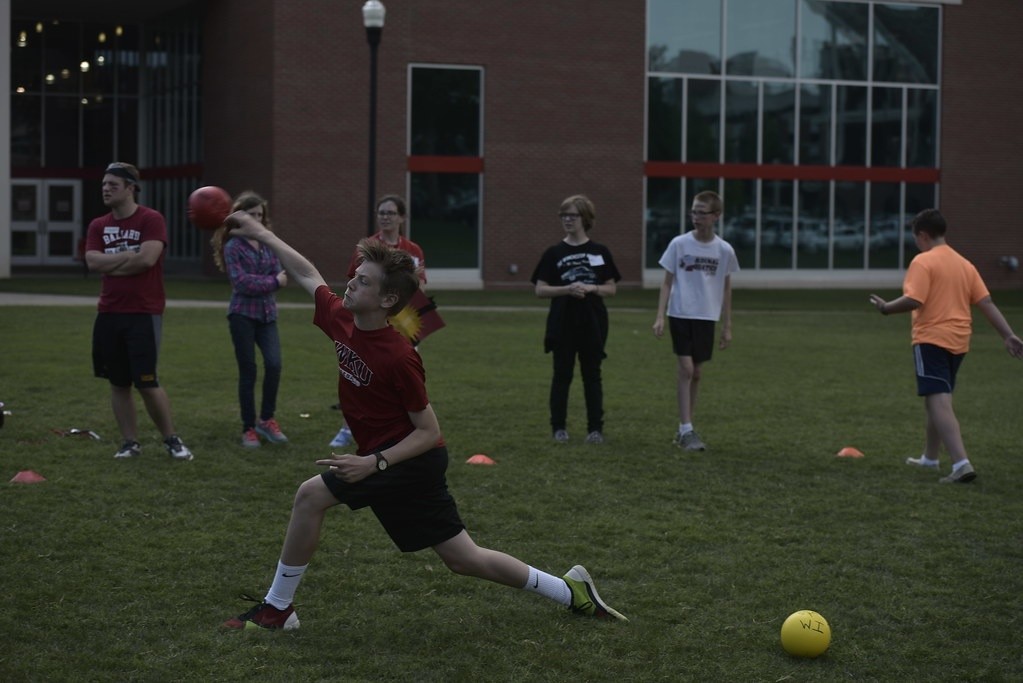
[728,211,913,255]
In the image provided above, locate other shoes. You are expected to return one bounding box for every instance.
[588,430,604,443]
[328,428,353,446]
[555,430,569,442]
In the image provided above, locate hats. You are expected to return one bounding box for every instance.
[106,162,140,193]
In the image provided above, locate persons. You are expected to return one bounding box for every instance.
[223,210,630,635]
[86,162,194,462]
[211,191,290,448]
[328,195,428,449]
[869,209,1023,483]
[530,194,621,443]
[654,191,740,454]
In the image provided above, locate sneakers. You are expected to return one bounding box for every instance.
[939,462,976,483]
[560,564,628,622]
[673,428,706,451]
[112,442,142,458]
[906,455,940,470]
[258,418,288,442]
[162,433,192,460]
[223,602,301,634]
[243,429,262,448]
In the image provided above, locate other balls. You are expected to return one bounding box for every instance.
[781,610,831,656]
[190,187,231,228]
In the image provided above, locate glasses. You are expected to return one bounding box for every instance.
[379,209,401,220]
[558,212,582,220]
[689,208,716,217]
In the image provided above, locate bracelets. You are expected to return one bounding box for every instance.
[881,302,887,316]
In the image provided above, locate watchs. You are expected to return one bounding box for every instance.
[373,451,388,472]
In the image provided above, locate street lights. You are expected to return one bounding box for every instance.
[362,0,387,238]
